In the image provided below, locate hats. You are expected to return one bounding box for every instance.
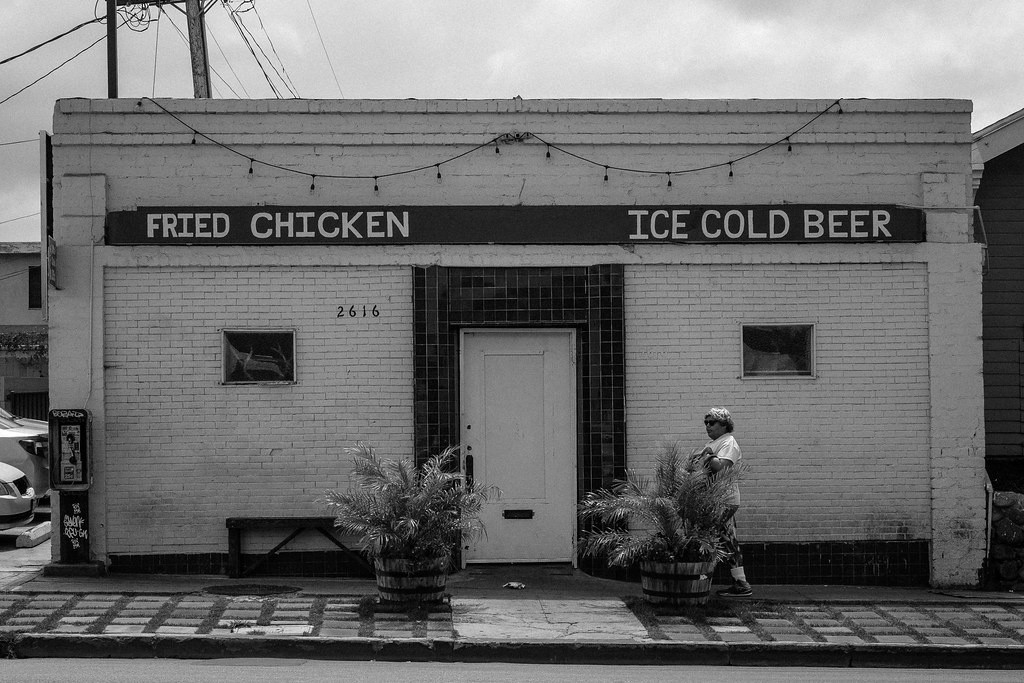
[705,406,734,425]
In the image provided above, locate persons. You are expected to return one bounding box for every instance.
[694,407,753,597]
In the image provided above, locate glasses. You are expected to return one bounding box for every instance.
[704,420,718,426]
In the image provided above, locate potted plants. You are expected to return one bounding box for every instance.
[573,434,750,611]
[328,443,500,612]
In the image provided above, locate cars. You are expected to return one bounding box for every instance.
[0,461,37,532]
[0,403,50,432]
[0,417,49,497]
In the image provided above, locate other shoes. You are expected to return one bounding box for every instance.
[716,579,752,596]
